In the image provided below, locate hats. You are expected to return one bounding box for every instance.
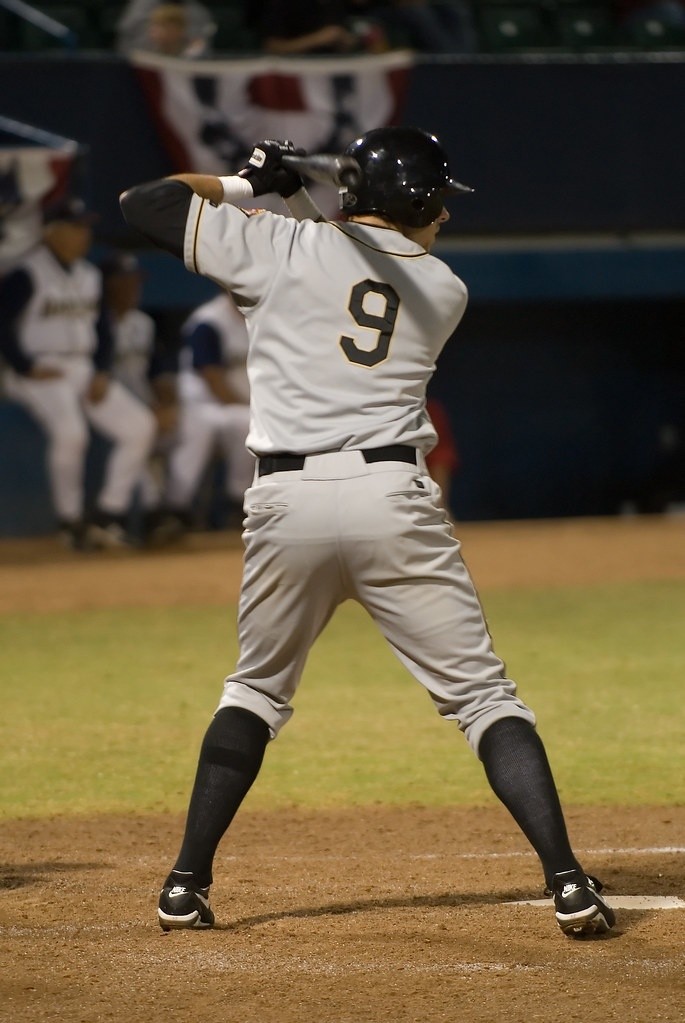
[43,195,104,224]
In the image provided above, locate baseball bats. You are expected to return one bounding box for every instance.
[281,153,364,190]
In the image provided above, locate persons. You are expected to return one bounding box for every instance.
[117,127,617,941]
[118,0,391,60]
[0,193,256,553]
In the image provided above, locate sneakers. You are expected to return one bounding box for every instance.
[157,870,216,928]
[544,874,614,936]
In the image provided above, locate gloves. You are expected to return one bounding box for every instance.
[236,139,306,198]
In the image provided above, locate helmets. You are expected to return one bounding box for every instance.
[340,126,476,228]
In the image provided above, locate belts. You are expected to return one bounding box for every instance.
[258,444,416,476]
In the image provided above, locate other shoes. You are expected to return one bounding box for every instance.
[65,514,181,552]
